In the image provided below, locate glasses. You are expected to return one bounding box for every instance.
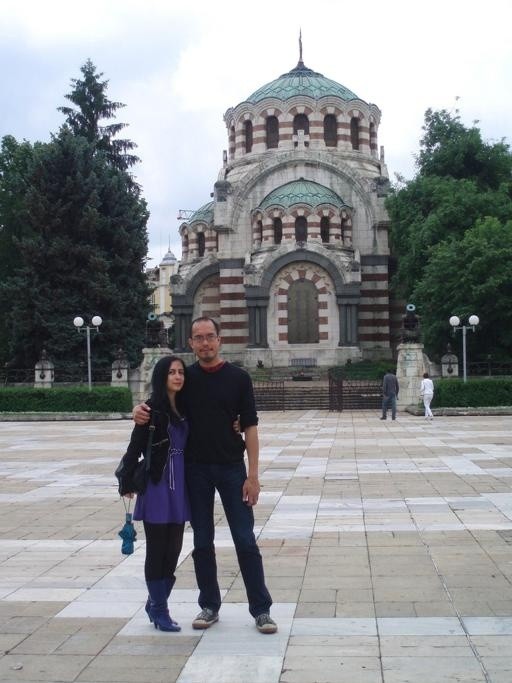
[193,335,216,341]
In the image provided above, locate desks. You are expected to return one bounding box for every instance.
[292,374,313,381]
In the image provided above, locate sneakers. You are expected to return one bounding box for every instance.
[255,613,277,633]
[192,607,219,629]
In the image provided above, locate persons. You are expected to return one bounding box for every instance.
[380,369,399,419]
[126,352,245,631]
[130,316,278,633]
[418,371,435,419]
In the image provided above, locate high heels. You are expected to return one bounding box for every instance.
[145,575,181,632]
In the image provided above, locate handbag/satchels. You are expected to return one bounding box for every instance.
[115,414,163,497]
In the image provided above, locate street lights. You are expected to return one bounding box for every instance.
[73,315,103,390]
[449,314,480,382]
